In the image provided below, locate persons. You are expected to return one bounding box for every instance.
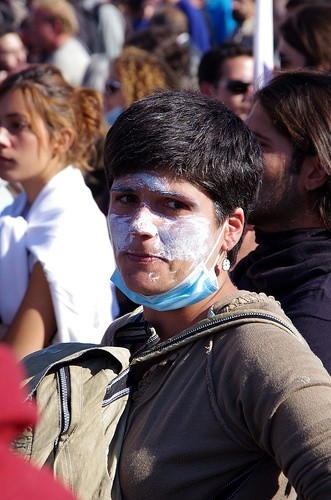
[236,72,331,374]
[1,0,331,126]
[101,87,331,500]
[0,64,116,376]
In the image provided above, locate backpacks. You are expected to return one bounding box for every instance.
[12,303,307,500]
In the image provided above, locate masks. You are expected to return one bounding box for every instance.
[110,215,226,313]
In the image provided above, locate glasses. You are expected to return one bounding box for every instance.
[105,79,122,93]
[221,79,254,92]
